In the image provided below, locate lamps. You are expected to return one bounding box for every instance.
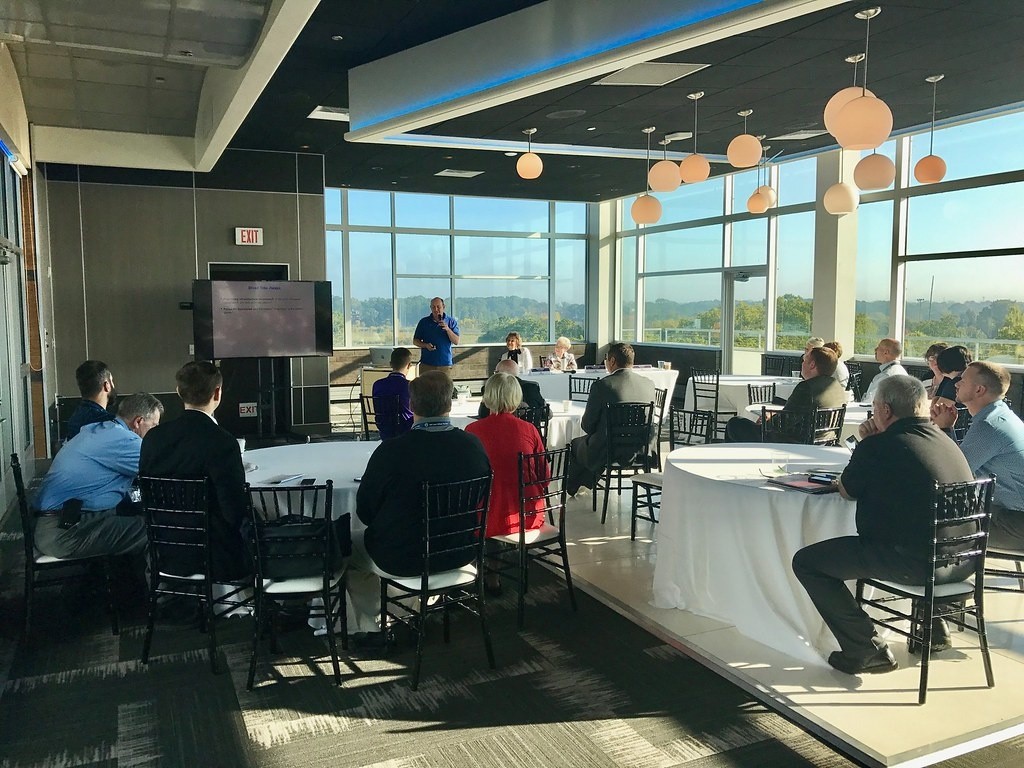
[516,127,543,180]
[632,8,947,224]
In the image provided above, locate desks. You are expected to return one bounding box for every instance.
[684,375,803,440]
[526,365,682,426]
[747,400,933,453]
[647,443,891,654]
[448,398,586,457]
[241,442,442,642]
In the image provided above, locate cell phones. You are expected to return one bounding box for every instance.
[808,476,832,486]
[300,478,316,486]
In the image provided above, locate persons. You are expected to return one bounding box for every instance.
[561,343,655,496]
[28,392,203,630]
[931,346,973,440]
[922,343,949,400]
[372,347,414,441]
[478,360,552,436]
[413,297,459,378]
[545,337,577,372]
[345,369,491,651]
[725,347,847,444]
[66,360,115,440]
[823,342,850,391]
[500,331,533,375]
[917,362,1024,618]
[793,375,981,675]
[860,338,908,403]
[799,338,825,380]
[466,372,551,609]
[139,360,310,633]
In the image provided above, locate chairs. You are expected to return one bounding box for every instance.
[11,453,121,650]
[359,394,400,441]
[569,356,1024,704]
[138,473,256,675]
[512,404,549,450]
[243,479,349,690]
[379,469,497,692]
[490,443,577,630]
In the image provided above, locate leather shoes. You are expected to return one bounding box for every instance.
[907,616,952,654]
[827,645,900,675]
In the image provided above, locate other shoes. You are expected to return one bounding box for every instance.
[483,574,501,596]
[149,605,206,631]
[355,630,397,652]
[564,465,579,496]
[255,612,303,631]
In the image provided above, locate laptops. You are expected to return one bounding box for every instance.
[843,434,859,454]
[369,348,394,366]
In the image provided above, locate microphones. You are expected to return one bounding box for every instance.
[563,370,576,374]
[438,314,443,330]
